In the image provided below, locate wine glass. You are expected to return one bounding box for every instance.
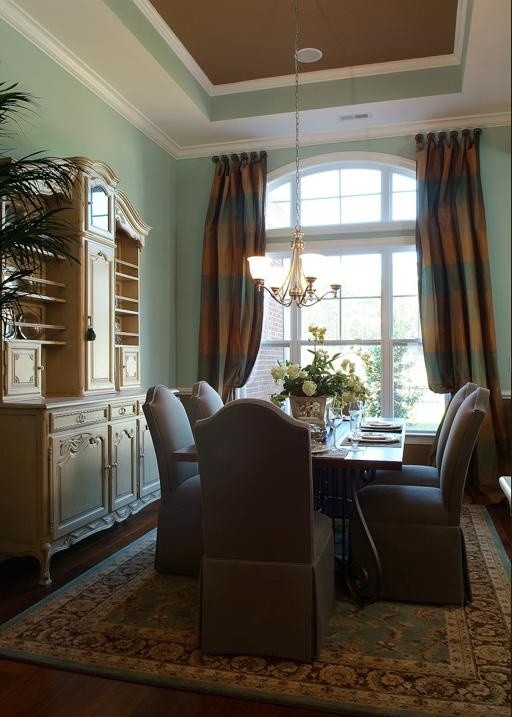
[326,407,345,453]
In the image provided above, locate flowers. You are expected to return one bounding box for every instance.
[269,322,377,413]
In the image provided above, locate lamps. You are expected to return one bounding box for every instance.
[245,1,344,308]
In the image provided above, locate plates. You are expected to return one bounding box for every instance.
[2,302,18,340]
[349,420,401,444]
[311,443,330,455]
[19,312,43,340]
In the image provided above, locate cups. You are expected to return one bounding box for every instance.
[348,401,363,441]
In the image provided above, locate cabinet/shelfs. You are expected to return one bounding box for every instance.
[0,406,139,587]
[138,394,165,513]
[115,191,153,390]
[3,158,67,399]
[48,157,115,403]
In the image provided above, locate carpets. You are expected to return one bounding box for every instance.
[1,500,509,716]
